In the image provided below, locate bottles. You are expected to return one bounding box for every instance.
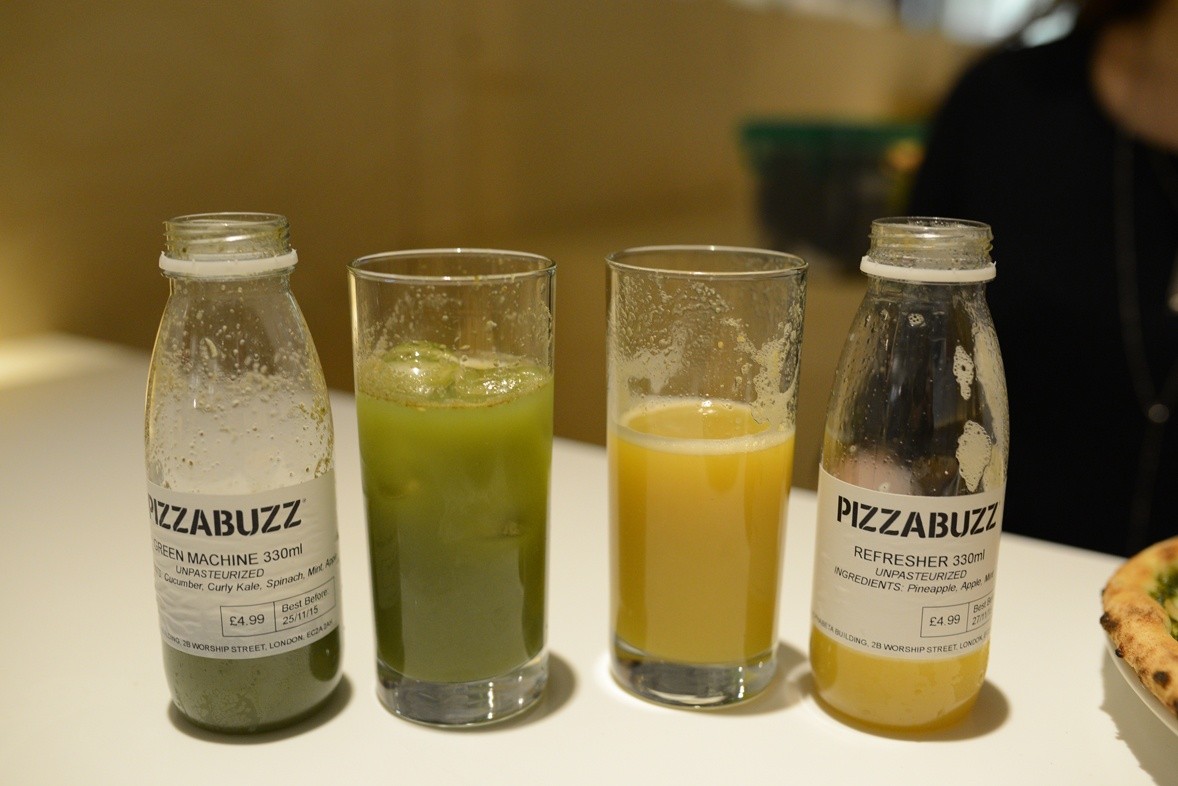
[141,212,342,735]
[810,215,1009,726]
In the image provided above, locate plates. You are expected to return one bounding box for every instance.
[1107,630,1178,736]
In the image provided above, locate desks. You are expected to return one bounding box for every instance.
[0,328,1157,786]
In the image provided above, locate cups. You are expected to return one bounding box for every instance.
[605,242,809,708]
[347,247,557,730]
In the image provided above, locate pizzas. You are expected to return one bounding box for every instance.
[1100,538,1178,716]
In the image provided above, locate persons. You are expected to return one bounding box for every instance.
[839,0,1178,560]
